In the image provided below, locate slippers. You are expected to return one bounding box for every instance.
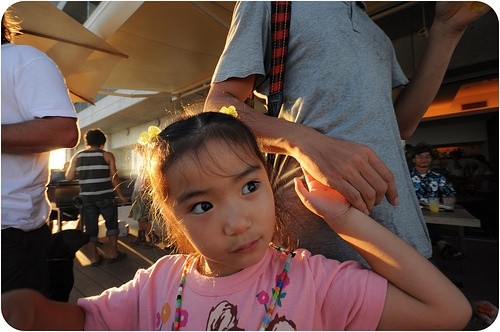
[108,251,127,263]
[90,254,103,266]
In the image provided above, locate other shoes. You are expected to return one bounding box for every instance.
[130,229,147,246]
[145,231,159,249]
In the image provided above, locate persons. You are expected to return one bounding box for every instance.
[461,155,488,202]
[1,11,82,303]
[207,1,491,271]
[130,138,161,249]
[408,144,457,254]
[66,129,128,267]
[446,152,469,176]
[1,110,473,331]
[430,157,441,172]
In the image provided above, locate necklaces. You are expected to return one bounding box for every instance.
[173,243,292,331]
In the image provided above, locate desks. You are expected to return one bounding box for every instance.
[422,209,481,252]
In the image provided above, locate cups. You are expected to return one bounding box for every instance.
[429,198,439,213]
[442,198,456,213]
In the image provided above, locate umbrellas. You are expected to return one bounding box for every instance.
[2,1,129,106]
